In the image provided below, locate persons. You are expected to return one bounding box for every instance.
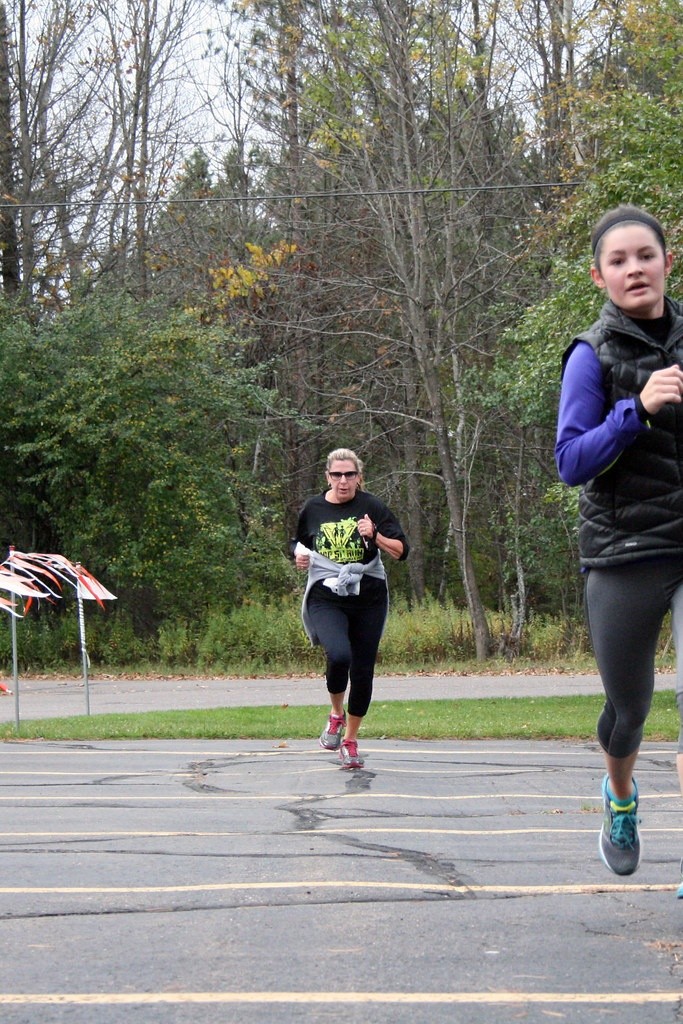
[556,208,682,899]
[295,448,411,767]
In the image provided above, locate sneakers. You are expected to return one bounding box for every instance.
[598,772,641,876]
[321,710,346,750]
[340,739,365,768]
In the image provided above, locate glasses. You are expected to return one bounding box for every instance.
[328,470,359,482]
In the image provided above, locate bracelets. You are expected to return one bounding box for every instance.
[371,524,379,542]
